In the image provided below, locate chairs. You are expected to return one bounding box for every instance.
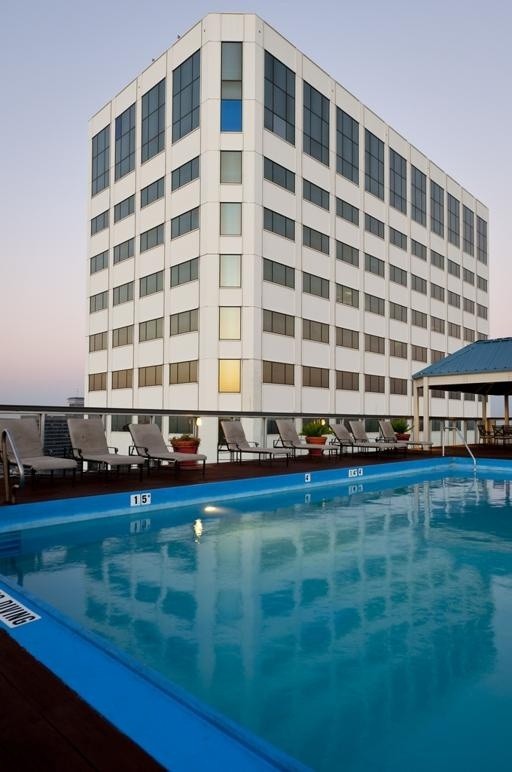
[217,418,432,467]
[1,417,209,494]
[476,423,511,449]
